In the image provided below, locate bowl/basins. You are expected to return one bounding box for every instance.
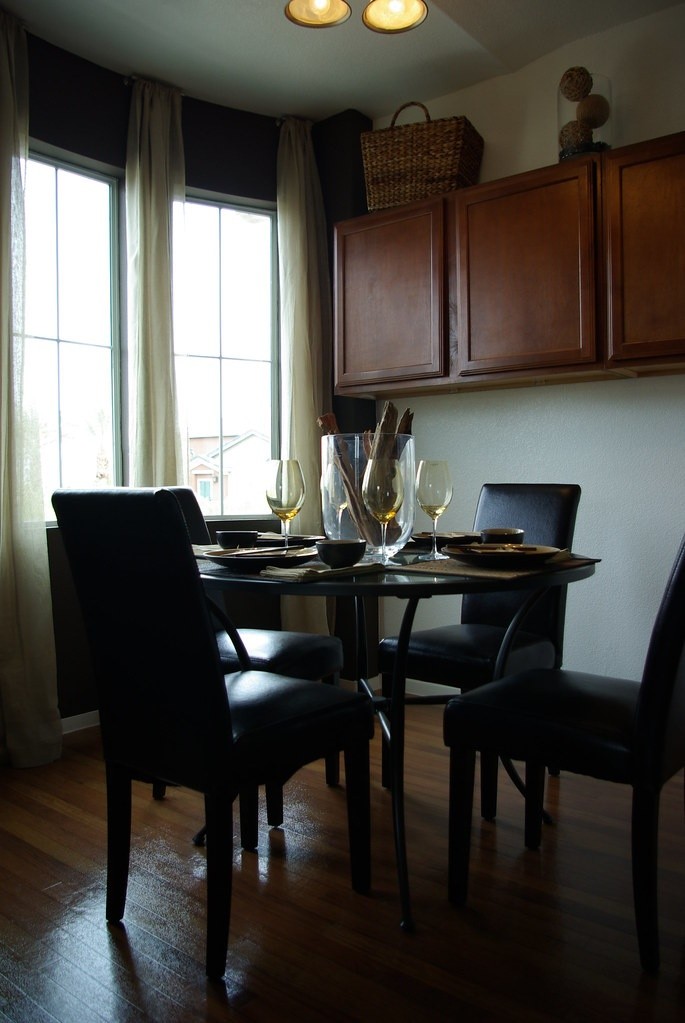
[216,531,258,550]
[480,528,524,544]
[440,544,561,568]
[316,539,366,569]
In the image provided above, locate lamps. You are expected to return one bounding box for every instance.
[283,0,352,29]
[361,0,429,34]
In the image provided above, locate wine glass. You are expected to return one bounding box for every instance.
[267,461,305,548]
[360,459,405,566]
[415,459,452,561]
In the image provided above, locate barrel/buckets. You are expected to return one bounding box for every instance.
[321,434,416,562]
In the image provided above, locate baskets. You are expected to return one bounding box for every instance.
[361,99,483,210]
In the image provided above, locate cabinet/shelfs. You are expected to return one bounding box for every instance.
[332,130,684,403]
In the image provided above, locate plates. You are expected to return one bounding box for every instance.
[204,548,318,571]
[411,527,479,547]
[256,534,326,547]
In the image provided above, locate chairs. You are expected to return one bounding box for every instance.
[442,533,685,970]
[171,488,342,829]
[52,490,374,980]
[376,483,582,820]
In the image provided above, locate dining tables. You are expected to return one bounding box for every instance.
[196,545,596,936]
